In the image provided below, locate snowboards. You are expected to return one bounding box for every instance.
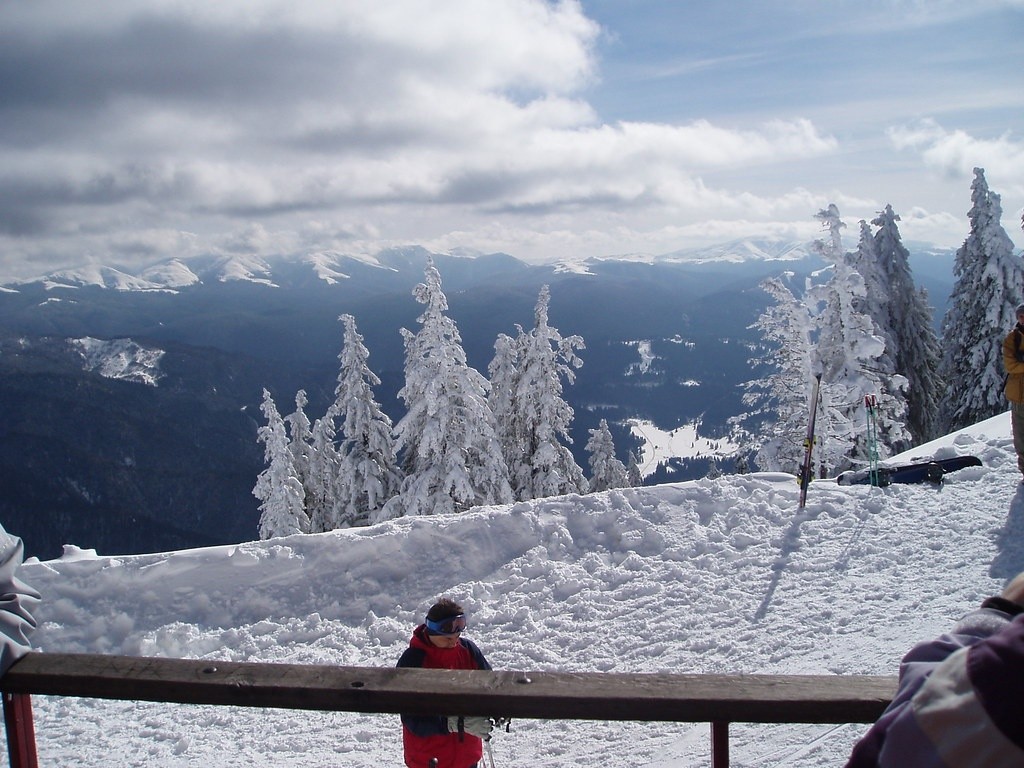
[836,455,984,488]
[798,371,822,509]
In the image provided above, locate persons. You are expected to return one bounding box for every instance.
[396,603,497,767]
[841,307,1024,768]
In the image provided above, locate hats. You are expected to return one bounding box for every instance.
[1016,303,1024,320]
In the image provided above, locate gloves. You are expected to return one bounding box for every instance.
[447,715,492,740]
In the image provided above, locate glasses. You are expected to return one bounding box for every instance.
[437,614,467,635]
